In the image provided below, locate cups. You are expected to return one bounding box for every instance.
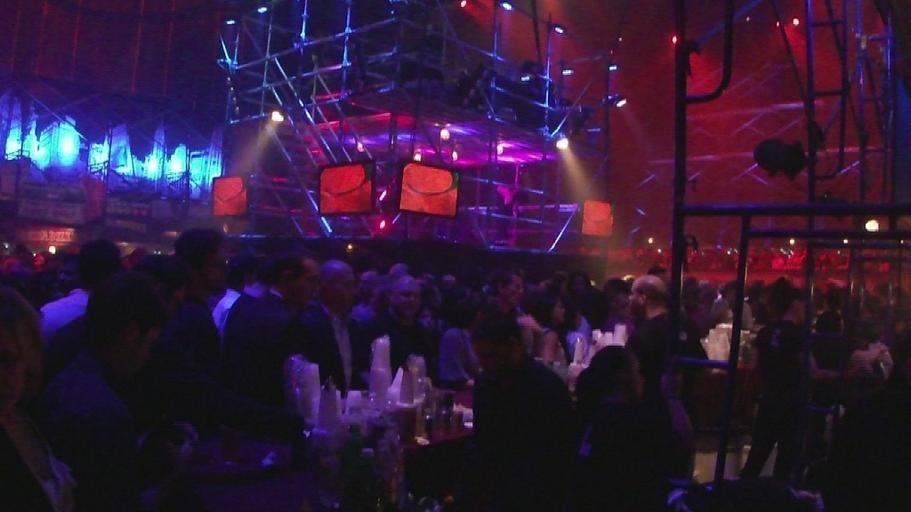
[286,333,426,444]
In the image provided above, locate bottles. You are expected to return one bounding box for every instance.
[353,448,384,512]
[569,360,585,396]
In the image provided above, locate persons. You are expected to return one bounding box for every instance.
[2,228,910,511]
